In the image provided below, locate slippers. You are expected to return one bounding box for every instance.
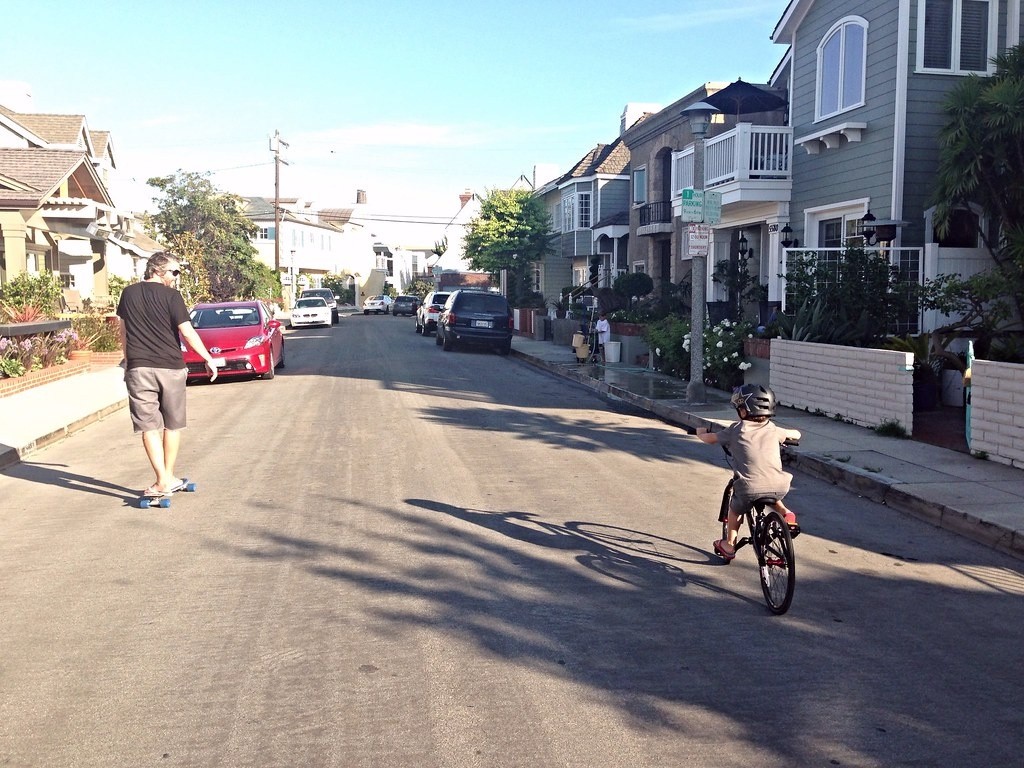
[782,513,797,533]
[172,478,184,489]
[713,539,736,559]
[144,485,171,497]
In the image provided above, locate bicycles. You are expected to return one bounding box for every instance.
[687,422,802,616]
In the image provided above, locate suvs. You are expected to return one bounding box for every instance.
[415,290,453,337]
[299,287,341,324]
[434,287,515,355]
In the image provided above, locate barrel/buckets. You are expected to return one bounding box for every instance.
[574,342,590,359]
[605,342,621,362]
[572,330,585,347]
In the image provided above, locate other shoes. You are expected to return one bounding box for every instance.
[589,348,600,354]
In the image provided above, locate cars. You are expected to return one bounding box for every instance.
[393,295,423,317]
[179,299,286,386]
[363,294,394,315]
[288,296,333,330]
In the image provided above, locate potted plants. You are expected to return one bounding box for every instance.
[742,325,773,357]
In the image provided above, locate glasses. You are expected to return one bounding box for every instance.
[160,268,183,277]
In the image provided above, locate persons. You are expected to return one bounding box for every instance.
[115,251,218,496]
[593,310,610,361]
[696,382,801,560]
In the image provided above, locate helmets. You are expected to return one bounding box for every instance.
[731,384,778,418]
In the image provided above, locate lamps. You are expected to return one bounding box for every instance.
[736,230,754,260]
[779,222,798,249]
[861,210,882,245]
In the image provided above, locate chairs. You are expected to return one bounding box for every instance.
[202,310,218,325]
[244,314,258,324]
